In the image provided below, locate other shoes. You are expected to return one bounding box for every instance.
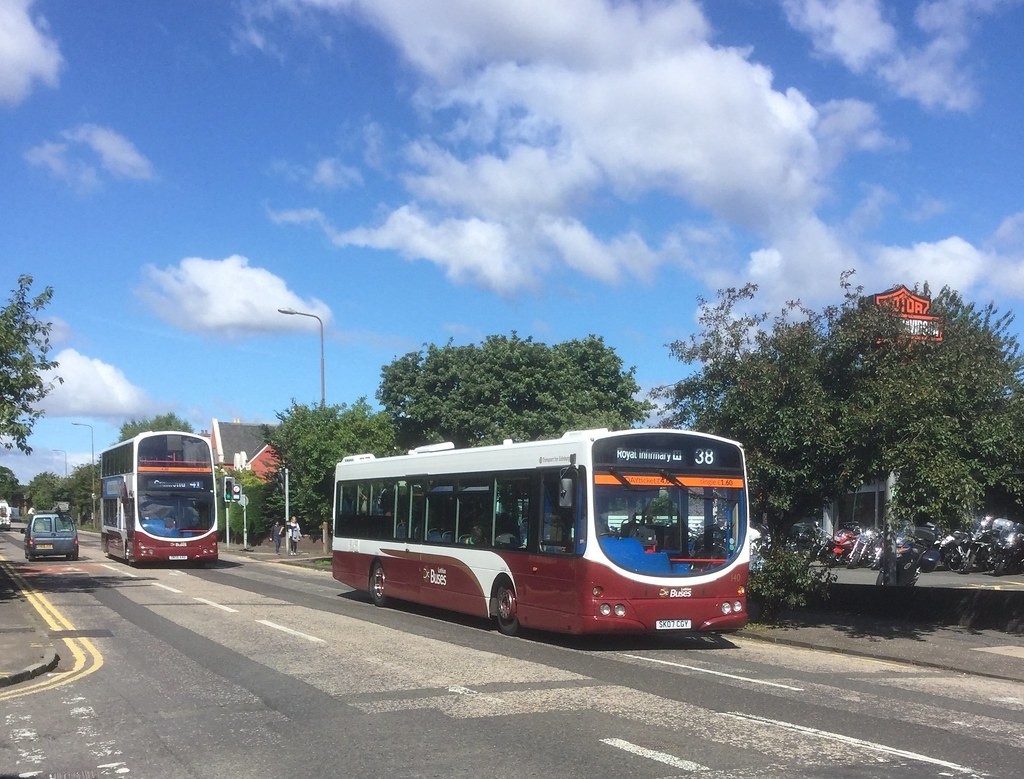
[290,551,293,555]
[278,552,280,555]
[294,552,296,555]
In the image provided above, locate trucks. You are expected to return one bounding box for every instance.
[0,500,12,530]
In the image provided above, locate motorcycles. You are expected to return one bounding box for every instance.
[829,516,1024,577]
[869,528,919,599]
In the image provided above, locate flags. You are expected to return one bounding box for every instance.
[245,444,284,479]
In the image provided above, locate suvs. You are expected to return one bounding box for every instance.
[20,512,79,561]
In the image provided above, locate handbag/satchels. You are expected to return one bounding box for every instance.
[268,535,274,542]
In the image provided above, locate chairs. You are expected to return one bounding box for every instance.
[396,522,471,545]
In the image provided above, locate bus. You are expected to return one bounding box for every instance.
[332,428,748,640]
[101,430,218,569]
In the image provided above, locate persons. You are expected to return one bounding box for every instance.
[288,515,302,556]
[269,519,282,555]
[378,480,395,516]
[465,524,487,547]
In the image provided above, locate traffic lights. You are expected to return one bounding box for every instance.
[226,481,231,500]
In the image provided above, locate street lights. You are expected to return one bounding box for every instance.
[52,450,67,478]
[278,307,330,555]
[72,422,96,530]
